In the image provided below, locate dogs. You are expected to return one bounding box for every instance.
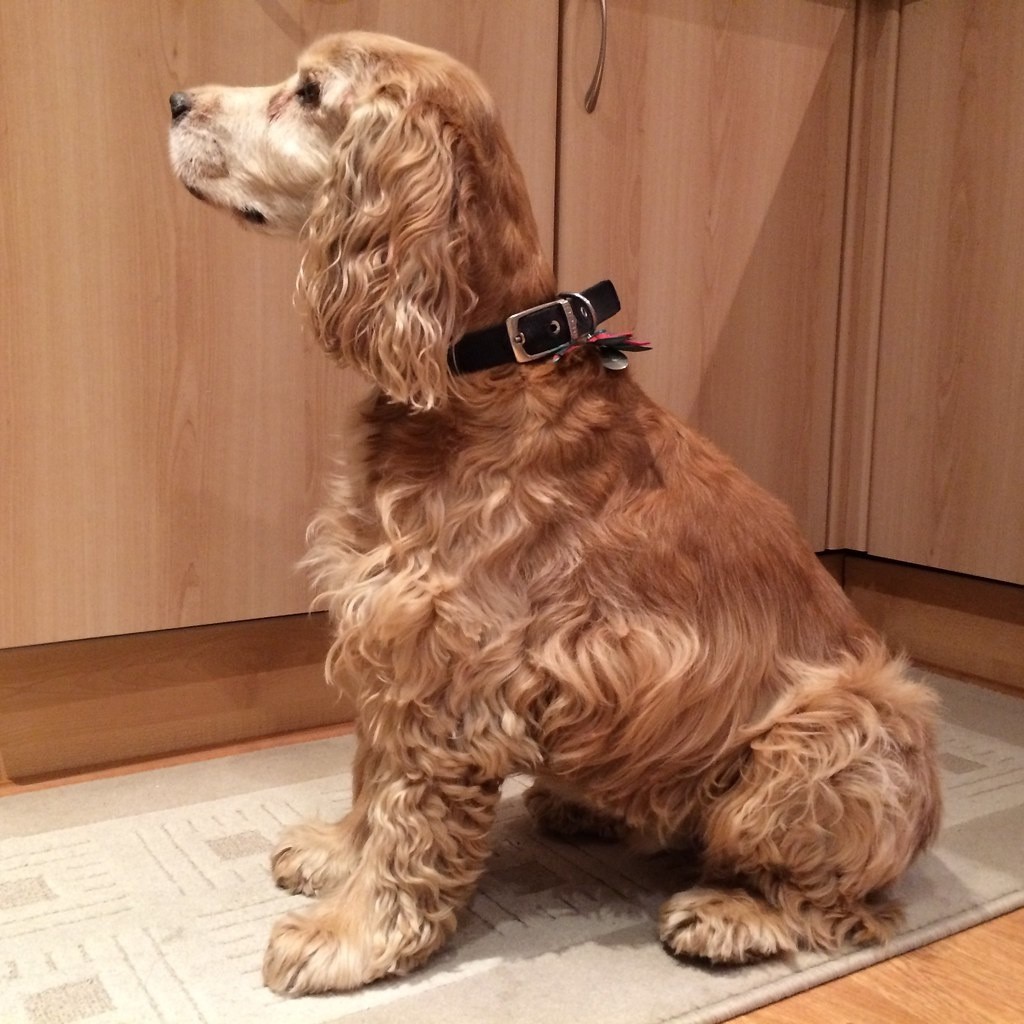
[168,29,945,997]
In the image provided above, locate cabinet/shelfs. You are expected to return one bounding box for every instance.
[0,0,1024,779]
